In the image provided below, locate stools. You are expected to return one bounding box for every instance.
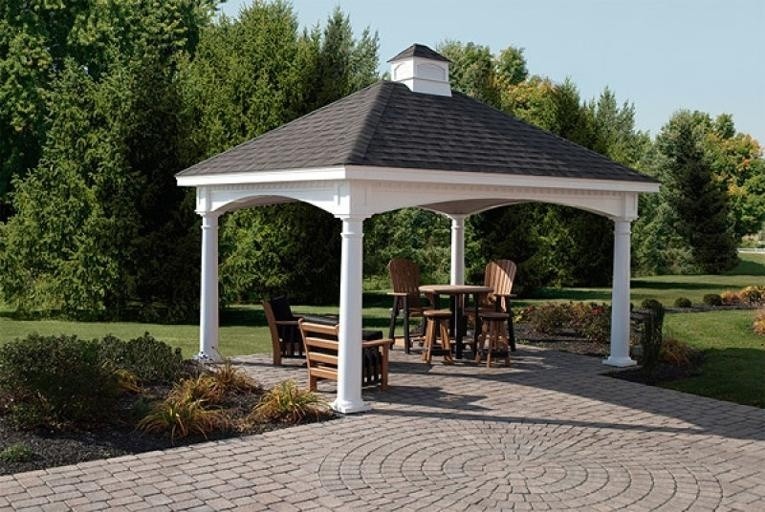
[423,308,453,364]
[474,312,511,368]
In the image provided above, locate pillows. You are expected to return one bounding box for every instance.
[363,330,383,366]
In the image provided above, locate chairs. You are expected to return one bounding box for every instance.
[385,257,427,354]
[464,260,517,352]
[262,294,338,366]
[298,317,393,391]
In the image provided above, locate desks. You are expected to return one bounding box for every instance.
[418,284,493,359]
